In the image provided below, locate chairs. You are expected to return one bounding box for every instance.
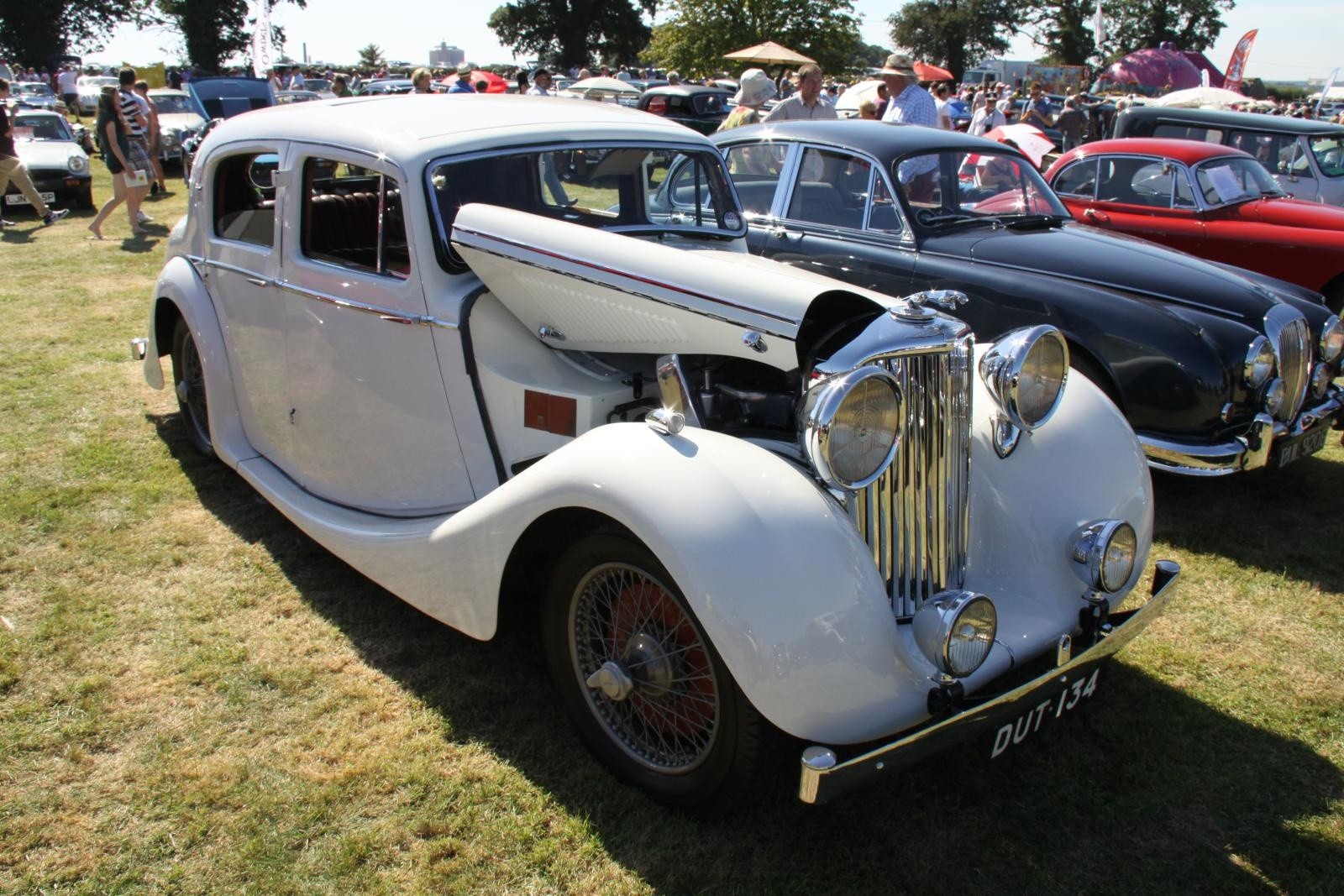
[766,199,839,225]
[859,205,921,230]
[329,244,409,270]
[37,127,55,135]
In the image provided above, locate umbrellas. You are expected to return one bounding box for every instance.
[723,41,817,64]
[913,60,953,81]
[958,122,1056,179]
[1156,83,1248,105]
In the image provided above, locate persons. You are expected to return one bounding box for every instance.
[1230,95,1344,163]
[407,63,488,94]
[305,65,334,81]
[716,54,937,203]
[492,67,515,80]
[52,64,82,124]
[0,58,51,82]
[0,77,69,227]
[571,64,683,85]
[85,66,168,240]
[351,62,411,91]
[267,66,307,102]
[518,67,578,207]
[335,75,354,98]
[917,74,1136,192]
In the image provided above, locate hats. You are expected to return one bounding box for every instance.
[877,54,924,78]
[457,63,472,77]
[734,68,777,105]
[985,92,998,101]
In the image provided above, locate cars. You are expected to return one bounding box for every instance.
[146,78,1097,160]
[1,109,91,211]
[2,81,66,115]
[611,119,1344,496]
[129,90,1183,810]
[76,75,119,112]
[969,136,1343,315]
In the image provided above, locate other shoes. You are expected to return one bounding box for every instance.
[567,198,578,205]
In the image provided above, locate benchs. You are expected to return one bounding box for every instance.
[236,189,497,252]
[676,181,847,226]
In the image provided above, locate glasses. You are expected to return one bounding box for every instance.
[1030,92,1041,96]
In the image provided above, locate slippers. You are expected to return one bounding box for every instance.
[133,230,153,234]
[86,235,107,240]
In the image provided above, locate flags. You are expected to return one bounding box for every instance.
[1094,1,1107,44]
[254,0,273,79]
[1224,28,1258,89]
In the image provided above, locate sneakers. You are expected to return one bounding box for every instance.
[0,219,15,226]
[44,209,69,226]
[136,211,154,222]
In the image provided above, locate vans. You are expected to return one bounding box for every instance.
[1113,105,1344,210]
